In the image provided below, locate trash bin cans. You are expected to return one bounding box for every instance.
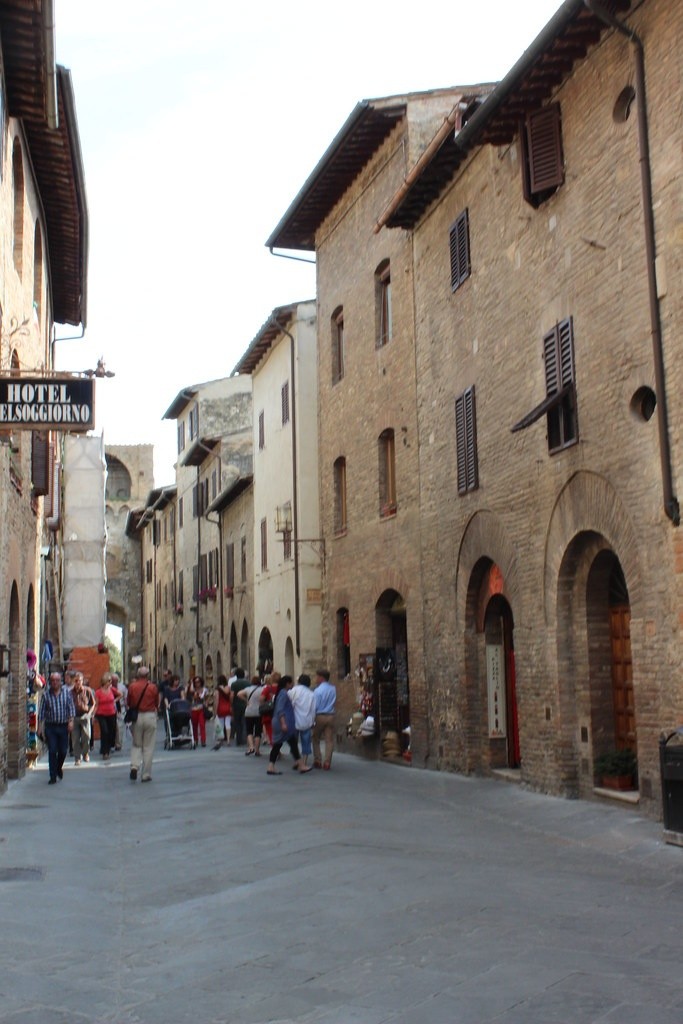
[659,731,683,845]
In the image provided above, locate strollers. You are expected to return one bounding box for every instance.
[164,699,197,751]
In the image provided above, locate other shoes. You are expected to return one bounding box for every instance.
[103,752,109,760]
[130,769,137,780]
[293,764,299,769]
[48,779,56,784]
[300,767,312,773]
[57,769,63,779]
[255,752,261,756]
[323,763,330,770]
[266,770,282,775]
[142,777,152,782]
[82,752,89,762]
[202,744,206,747]
[245,748,254,755]
[313,762,322,769]
[75,760,80,765]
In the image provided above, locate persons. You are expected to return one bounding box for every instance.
[211,666,266,757]
[156,670,211,747]
[259,670,337,775]
[94,672,128,760]
[37,673,75,784]
[126,667,161,783]
[62,670,95,766]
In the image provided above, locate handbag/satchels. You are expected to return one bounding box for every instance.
[124,709,138,724]
[213,714,225,741]
[259,701,274,715]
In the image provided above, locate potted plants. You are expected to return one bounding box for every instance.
[601,746,637,790]
